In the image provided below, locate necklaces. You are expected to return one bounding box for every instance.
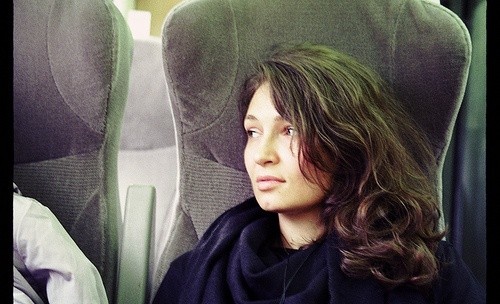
[280,232,328,304]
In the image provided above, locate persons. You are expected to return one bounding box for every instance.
[13,184,109,304]
[151,42,483,304]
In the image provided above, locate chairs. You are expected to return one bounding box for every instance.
[14,0,473,304]
